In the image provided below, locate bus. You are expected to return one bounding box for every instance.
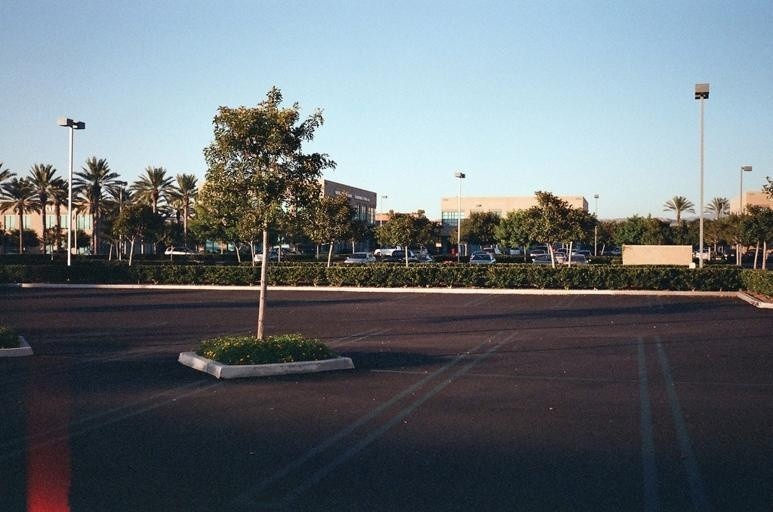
[251,247,302,266]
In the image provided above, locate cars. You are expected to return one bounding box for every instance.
[693,247,773,265]
[341,243,622,265]
[164,247,202,259]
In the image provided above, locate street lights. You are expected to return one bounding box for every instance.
[739,163,753,218]
[593,194,598,255]
[379,195,387,229]
[691,82,710,267]
[56,116,85,267]
[454,172,465,259]
